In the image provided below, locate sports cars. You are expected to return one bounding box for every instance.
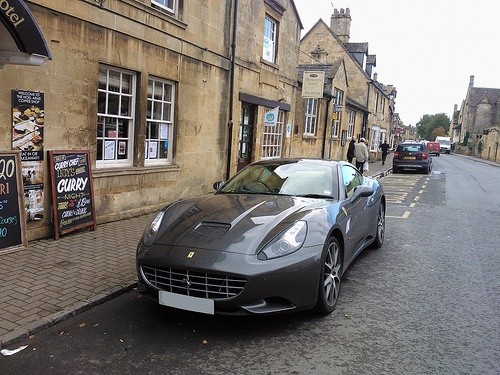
[136,155,386,317]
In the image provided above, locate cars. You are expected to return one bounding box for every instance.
[403,140,429,151]
[98,122,124,147]
[393,143,432,174]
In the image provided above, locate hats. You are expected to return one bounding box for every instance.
[360,138,366,142]
[352,136,357,142]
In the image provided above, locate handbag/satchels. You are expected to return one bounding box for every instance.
[363,160,370,171]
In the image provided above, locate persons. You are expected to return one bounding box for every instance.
[364,139,370,147]
[393,141,397,152]
[354,137,368,174]
[378,142,382,151]
[378,140,389,165]
[347,137,356,164]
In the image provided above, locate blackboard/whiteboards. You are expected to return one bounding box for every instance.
[0,149,29,256]
[46,149,97,242]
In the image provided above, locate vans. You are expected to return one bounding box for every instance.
[435,136,452,154]
[427,142,440,156]
